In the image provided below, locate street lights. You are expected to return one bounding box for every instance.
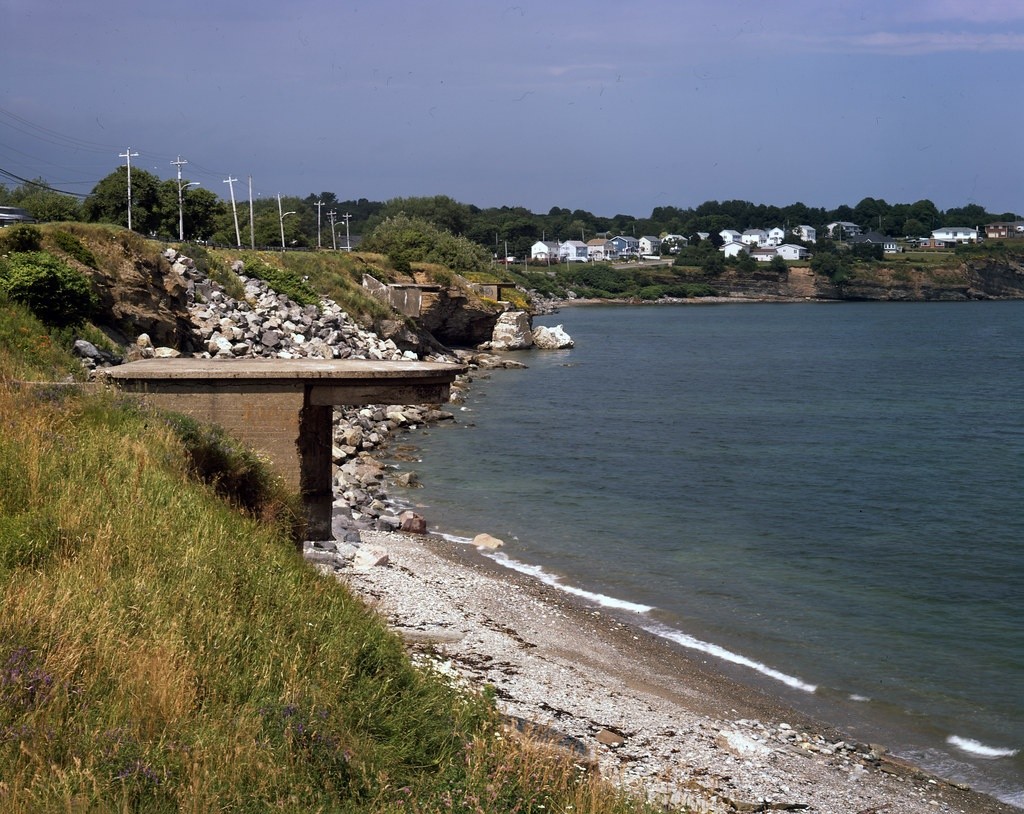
[178,181,201,244]
[332,221,345,251]
[280,211,297,247]
